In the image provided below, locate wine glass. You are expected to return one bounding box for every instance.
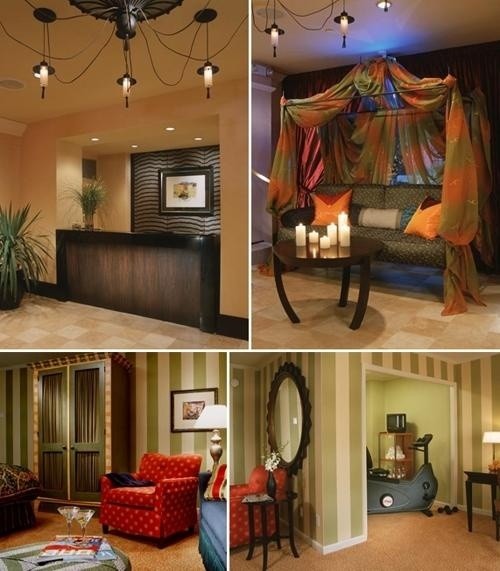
[73,510,96,547]
[58,507,79,544]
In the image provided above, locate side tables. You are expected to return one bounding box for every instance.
[240,491,299,571]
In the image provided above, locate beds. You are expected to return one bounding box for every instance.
[273,235,387,332]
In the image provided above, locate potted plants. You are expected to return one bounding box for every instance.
[0,202,56,312]
[55,175,116,229]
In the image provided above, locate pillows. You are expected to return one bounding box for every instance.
[308,188,351,226]
[202,462,228,502]
[359,204,405,230]
[402,192,447,240]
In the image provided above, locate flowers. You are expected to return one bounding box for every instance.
[259,441,291,474]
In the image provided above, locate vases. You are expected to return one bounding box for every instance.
[265,469,278,496]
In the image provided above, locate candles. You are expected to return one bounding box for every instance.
[320,245,338,259]
[309,244,319,253]
[296,246,307,259]
[339,247,350,258]
[292,211,352,249]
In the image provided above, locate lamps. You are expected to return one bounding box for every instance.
[480,431,500,471]
[255,1,398,61]
[192,405,228,480]
[29,1,221,111]
[157,165,214,217]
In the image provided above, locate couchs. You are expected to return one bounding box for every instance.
[265,180,470,273]
[230,464,295,553]
[99,452,202,547]
[0,462,40,537]
[198,498,226,570]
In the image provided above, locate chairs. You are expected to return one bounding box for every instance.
[367,447,399,483]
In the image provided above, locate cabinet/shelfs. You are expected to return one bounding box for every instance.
[377,432,416,484]
[38,362,106,505]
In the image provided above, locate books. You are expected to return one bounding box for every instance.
[37,536,103,558]
[58,538,117,561]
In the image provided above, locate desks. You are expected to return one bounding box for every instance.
[465,469,499,541]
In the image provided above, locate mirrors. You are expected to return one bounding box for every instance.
[265,361,313,476]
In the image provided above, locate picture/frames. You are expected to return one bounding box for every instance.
[169,387,220,435]
